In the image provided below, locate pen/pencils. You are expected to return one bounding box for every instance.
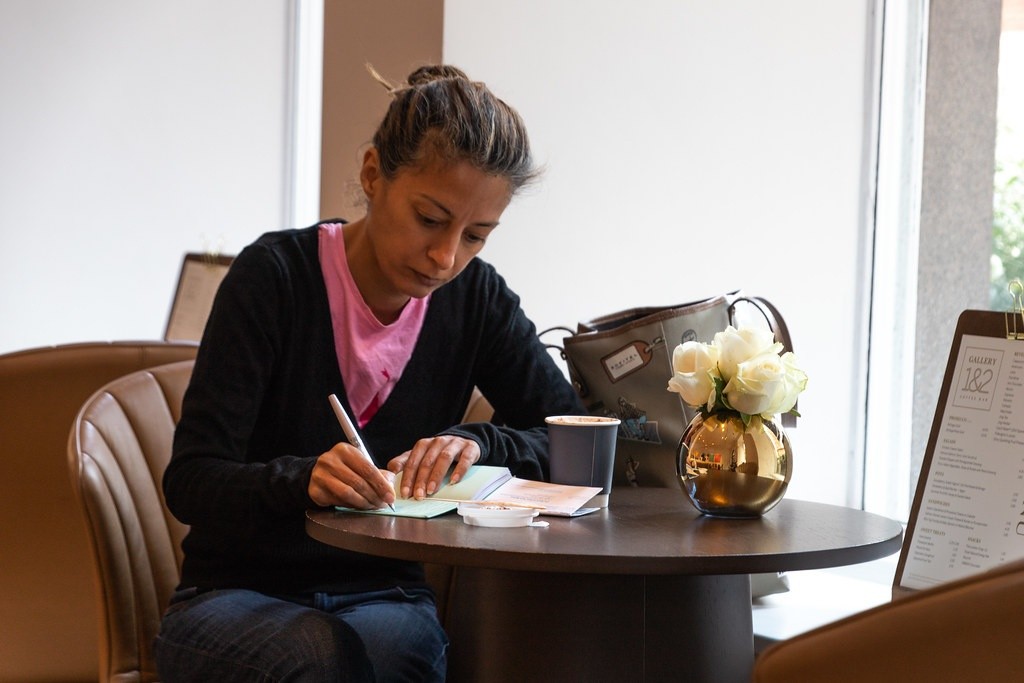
[328,393,396,512]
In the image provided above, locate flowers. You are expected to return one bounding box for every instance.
[667,328,817,424]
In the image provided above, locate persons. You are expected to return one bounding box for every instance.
[155,66,575,683]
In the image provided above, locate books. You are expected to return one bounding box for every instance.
[334,464,512,520]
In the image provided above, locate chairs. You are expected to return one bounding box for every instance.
[754,558,1024,683]
[66,360,199,683]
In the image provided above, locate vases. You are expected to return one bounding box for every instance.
[676,406,797,521]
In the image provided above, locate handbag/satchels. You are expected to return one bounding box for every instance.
[537,289,794,487]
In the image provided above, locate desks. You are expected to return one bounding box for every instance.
[304,490,900,683]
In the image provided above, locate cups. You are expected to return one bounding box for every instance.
[545,415,622,508]
